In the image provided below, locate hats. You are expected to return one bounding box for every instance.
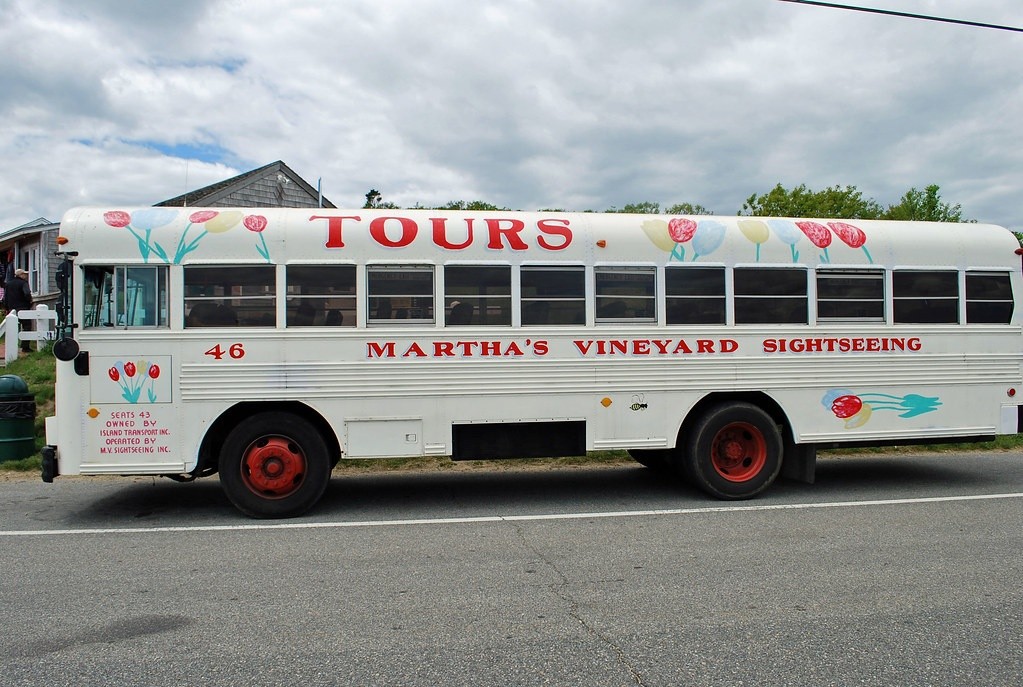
[14,269,29,275]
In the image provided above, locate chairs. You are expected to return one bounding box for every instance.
[183,294,777,325]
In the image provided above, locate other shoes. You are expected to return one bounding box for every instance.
[21,347,33,352]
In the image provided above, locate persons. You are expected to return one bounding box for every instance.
[2,269,34,352]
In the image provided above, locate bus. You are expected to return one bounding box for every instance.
[37,203,1023,521]
